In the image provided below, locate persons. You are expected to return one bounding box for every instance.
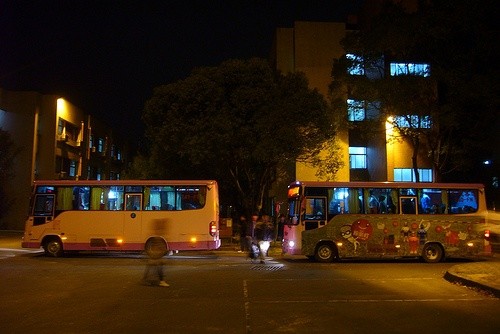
[39,186,473,287]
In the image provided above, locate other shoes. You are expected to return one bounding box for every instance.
[236,250,247,254]
[259,260,265,265]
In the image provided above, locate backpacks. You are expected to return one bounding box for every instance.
[249,243,260,259]
